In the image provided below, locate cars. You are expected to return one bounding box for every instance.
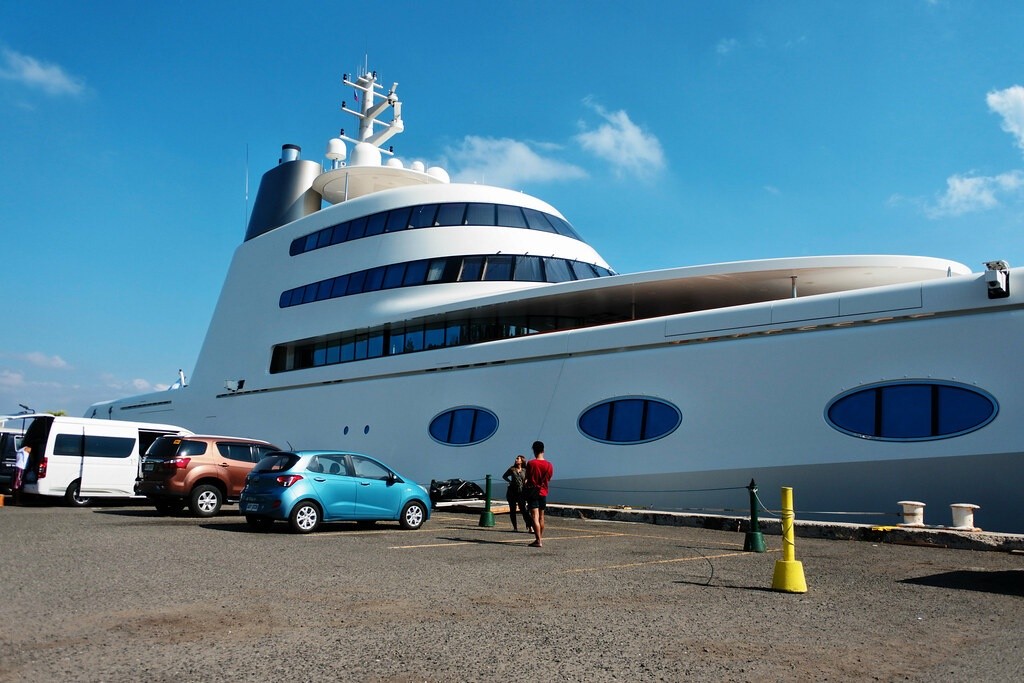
[238,450,433,535]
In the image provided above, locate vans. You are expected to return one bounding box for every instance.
[0,413,194,507]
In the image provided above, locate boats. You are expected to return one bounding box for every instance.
[80,51,1024,533]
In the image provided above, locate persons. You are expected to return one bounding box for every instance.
[502,455,533,535]
[12,445,31,506]
[523,440,553,547]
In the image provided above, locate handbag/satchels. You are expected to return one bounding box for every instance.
[521,484,540,499]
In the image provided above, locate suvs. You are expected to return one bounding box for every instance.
[134,434,282,517]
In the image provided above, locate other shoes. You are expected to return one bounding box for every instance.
[529,542,543,547]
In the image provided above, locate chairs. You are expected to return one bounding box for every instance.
[312,463,325,473]
[329,463,341,475]
